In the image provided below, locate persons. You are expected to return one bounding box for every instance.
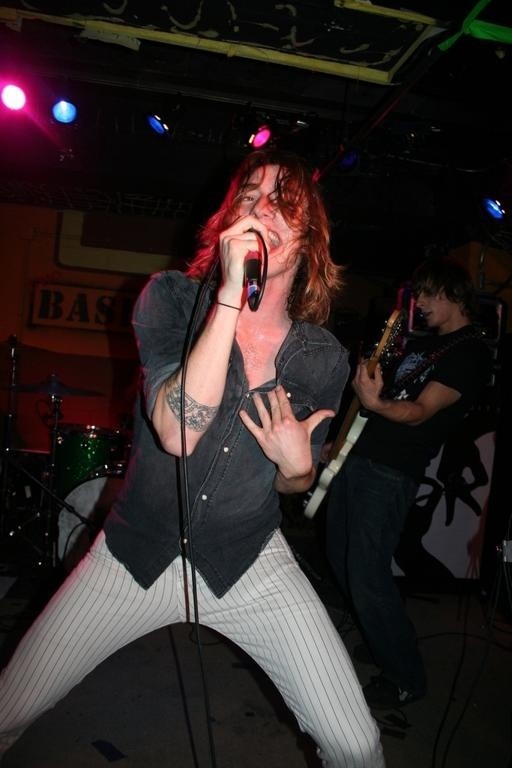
[0,148,389,768]
[318,262,483,708]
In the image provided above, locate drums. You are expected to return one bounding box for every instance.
[1,424,132,569]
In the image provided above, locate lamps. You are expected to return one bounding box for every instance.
[144,95,274,149]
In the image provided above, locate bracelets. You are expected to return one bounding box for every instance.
[215,302,242,312]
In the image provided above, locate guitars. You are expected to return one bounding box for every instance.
[301,307,408,519]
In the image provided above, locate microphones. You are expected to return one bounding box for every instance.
[244,228,261,311]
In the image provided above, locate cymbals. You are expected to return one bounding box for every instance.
[12,380,102,396]
